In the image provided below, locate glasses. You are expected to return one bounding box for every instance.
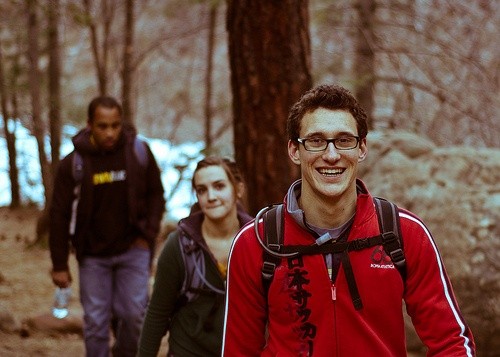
[297,135,362,153]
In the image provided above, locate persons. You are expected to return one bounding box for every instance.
[137,157,255,357]
[47,94,167,357]
[221,84,477,357]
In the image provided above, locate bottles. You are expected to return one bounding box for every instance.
[53,287,71,319]
